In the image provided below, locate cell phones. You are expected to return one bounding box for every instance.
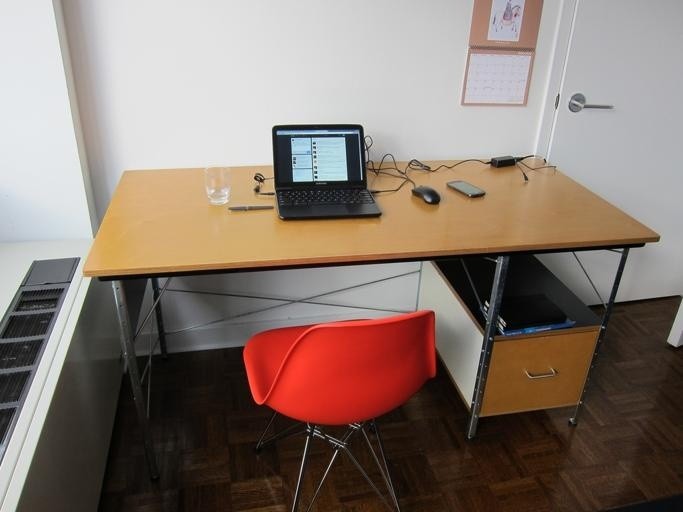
[447,179,487,198]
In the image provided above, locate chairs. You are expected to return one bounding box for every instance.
[240,310,437,512]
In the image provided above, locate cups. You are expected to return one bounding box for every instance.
[204,166,231,206]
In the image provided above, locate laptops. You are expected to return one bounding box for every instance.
[272,124,382,219]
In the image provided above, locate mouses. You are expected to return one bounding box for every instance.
[411,184,441,204]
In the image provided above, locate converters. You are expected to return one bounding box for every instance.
[490,155,516,168]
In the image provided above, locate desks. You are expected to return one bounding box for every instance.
[82,161,660,480]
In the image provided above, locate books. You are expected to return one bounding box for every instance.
[484,292,577,338]
